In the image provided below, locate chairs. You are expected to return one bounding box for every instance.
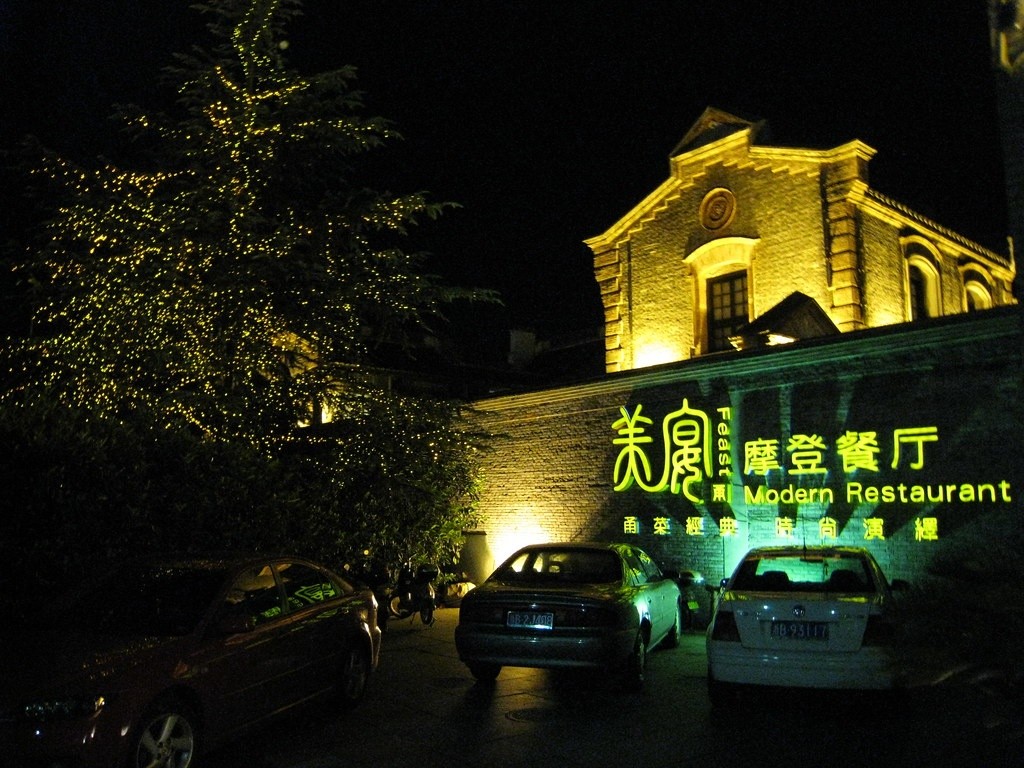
[542,559,564,582]
[831,569,862,593]
[763,571,792,591]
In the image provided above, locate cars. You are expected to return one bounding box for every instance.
[0,552,381,768]
[703,540,913,705]
[455,538,683,690]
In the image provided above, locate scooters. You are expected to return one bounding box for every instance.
[393,551,440,628]
